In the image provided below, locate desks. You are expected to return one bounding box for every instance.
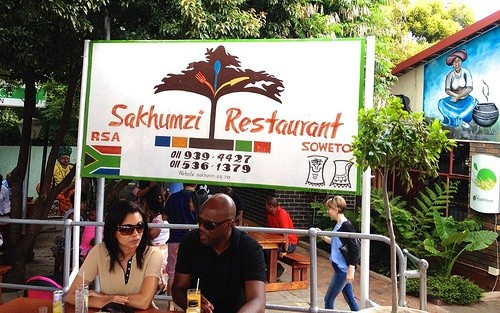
[0,297,187,313]
[247,230,287,283]
[52,246,83,274]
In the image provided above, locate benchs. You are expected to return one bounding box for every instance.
[277,250,311,282]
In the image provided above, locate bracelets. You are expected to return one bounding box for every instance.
[283,250,288,252]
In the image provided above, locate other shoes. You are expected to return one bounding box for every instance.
[276,263,285,280]
[174,308,178,312]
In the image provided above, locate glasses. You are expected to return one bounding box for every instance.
[117,221,145,235]
[195,215,232,230]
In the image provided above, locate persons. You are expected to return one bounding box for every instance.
[263,196,297,280]
[0,172,266,313]
[316,196,361,312]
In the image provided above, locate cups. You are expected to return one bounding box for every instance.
[53,290,65,313]
[244,224,248,233]
[75,284,88,313]
[186,289,201,313]
[39,307,48,313]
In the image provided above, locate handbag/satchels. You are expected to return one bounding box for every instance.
[339,236,361,268]
